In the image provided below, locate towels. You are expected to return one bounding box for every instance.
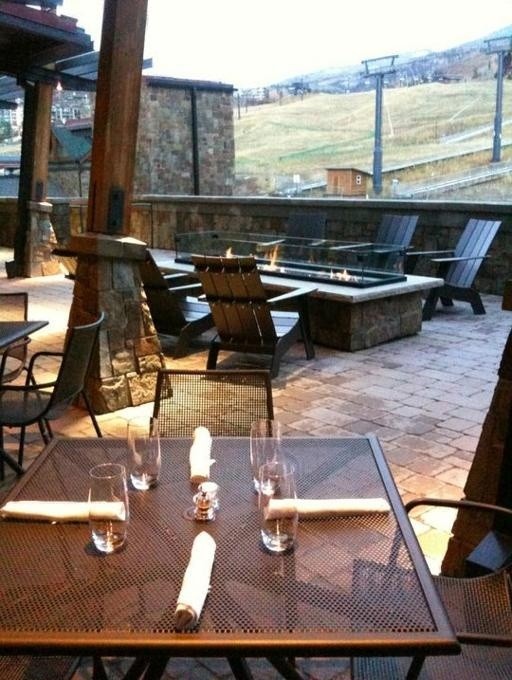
[1,500,126,523]
[173,528,218,630]
[189,425,215,484]
[264,498,390,520]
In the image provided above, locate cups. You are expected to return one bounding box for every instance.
[127,417,162,491]
[87,462,128,554]
[249,417,300,553]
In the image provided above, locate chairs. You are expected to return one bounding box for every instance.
[261,212,326,262]
[0,313,108,478]
[405,219,502,322]
[189,253,320,377]
[140,251,216,359]
[0,292,36,387]
[385,497,512,680]
[52,249,189,288]
[329,214,419,273]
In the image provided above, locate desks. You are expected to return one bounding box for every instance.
[156,254,445,351]
